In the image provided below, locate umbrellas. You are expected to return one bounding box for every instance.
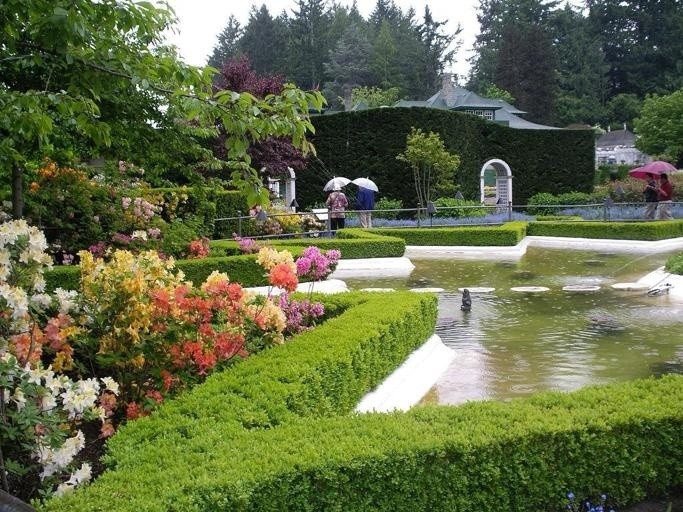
[643,161,677,174]
[351,176,378,192]
[323,175,351,190]
[628,167,645,179]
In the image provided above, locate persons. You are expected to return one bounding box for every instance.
[324,187,348,235]
[642,173,658,220]
[357,188,374,228]
[656,173,674,220]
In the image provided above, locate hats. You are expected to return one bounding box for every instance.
[333,184,342,191]
